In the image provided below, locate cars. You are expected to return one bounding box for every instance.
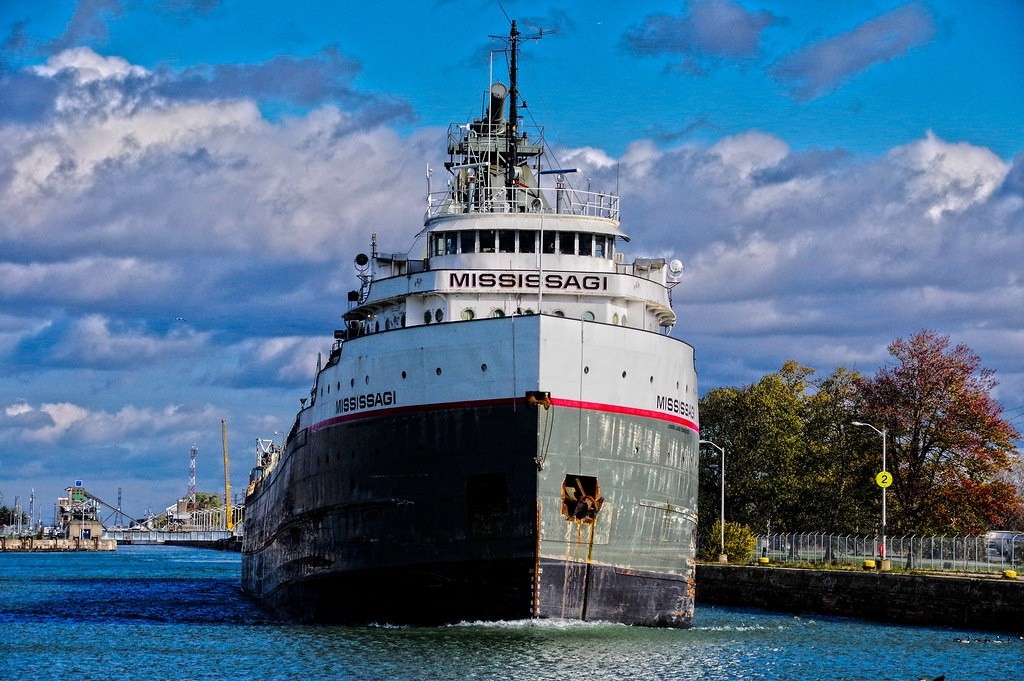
[769,539,791,551]
[988,530,1024,566]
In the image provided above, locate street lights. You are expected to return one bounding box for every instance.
[851,421,890,571]
[699,439,727,565]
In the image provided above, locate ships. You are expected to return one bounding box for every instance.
[240,1,700,629]
